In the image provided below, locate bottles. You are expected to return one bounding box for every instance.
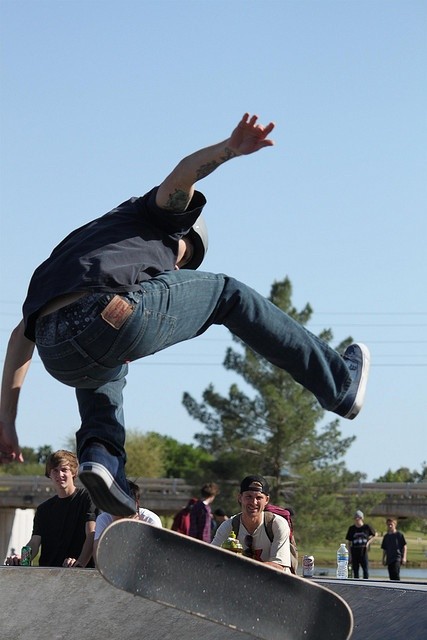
[336,544,349,577]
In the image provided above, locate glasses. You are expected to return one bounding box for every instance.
[244,532,253,556]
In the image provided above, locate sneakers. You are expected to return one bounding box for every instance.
[77,445,139,517]
[332,342,371,421]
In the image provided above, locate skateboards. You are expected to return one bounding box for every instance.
[95,518,355,639]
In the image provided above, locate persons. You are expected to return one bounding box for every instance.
[190,485,220,544]
[345,509,378,579]
[213,505,229,522]
[171,498,199,536]
[0,111,372,514]
[208,475,294,575]
[380,517,408,580]
[92,480,162,568]
[6,450,97,568]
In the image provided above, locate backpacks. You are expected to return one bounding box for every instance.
[232,503,299,575]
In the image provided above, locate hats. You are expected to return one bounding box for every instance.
[189,497,198,504]
[354,509,364,519]
[215,508,226,516]
[240,474,270,496]
[284,507,295,516]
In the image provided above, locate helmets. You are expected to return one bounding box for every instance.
[179,212,209,270]
